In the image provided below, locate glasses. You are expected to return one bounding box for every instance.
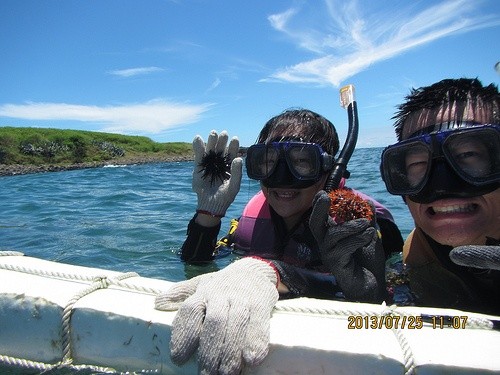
[379,125,500,195]
[246,141,334,181]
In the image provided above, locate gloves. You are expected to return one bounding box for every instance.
[191,130,243,217]
[154,254,280,375]
[449,244,500,269]
[309,190,377,303]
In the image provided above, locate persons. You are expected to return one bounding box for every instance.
[307,78,500,318]
[154,107,405,375]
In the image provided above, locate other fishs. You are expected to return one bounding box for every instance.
[197,149,233,186]
[328,188,373,224]
